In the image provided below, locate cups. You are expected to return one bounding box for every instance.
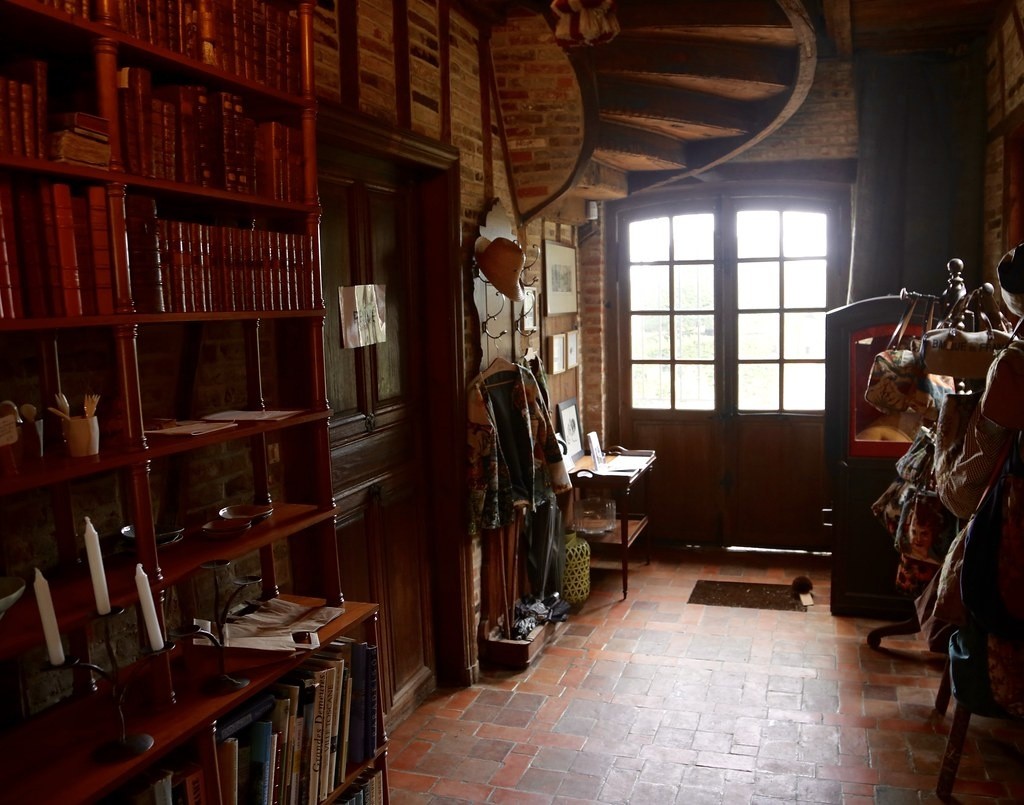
[63,416,99,457]
[573,498,616,534]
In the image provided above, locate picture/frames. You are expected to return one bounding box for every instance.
[542,239,579,316]
[564,329,579,370]
[548,333,567,375]
[556,397,585,463]
[521,286,538,331]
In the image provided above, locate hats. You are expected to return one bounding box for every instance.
[996,243,1024,294]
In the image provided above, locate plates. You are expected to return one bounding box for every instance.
[119,522,185,553]
[201,503,273,540]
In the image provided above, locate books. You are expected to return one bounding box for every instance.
[124,195,317,313]
[43,0,301,96]
[0,178,116,319]
[116,66,305,202]
[143,420,239,436]
[102,634,384,805]
[0,58,113,171]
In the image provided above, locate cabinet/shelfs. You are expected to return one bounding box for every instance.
[0,1,389,805]
[566,445,657,599]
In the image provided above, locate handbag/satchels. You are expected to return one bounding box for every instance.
[859,290,994,654]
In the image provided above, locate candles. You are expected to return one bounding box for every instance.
[84,515,111,616]
[133,563,166,651]
[33,567,65,666]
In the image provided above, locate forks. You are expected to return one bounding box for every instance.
[84,394,100,417]
[55,394,69,415]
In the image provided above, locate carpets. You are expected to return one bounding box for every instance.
[686,579,808,612]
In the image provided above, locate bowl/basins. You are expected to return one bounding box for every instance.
[0,578,26,615]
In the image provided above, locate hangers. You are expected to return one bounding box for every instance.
[482,336,520,389]
